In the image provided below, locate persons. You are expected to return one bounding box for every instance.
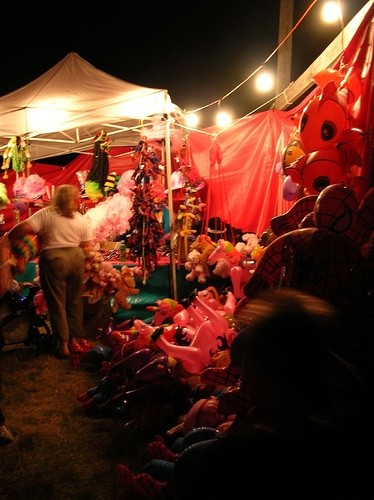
[5,184,99,356]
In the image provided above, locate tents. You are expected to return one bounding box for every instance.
[0,54,182,308]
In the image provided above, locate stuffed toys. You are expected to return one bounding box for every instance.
[182,230,266,286]
[81,247,142,315]
